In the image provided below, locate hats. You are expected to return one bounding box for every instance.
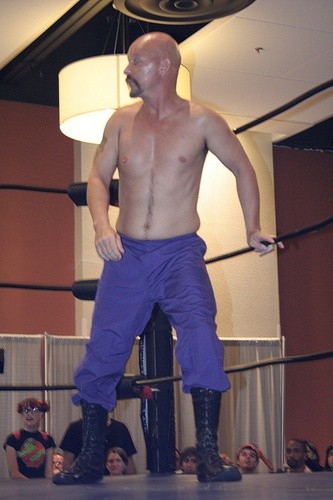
[304,440,320,459]
[241,443,261,458]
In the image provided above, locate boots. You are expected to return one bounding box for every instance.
[52,398,108,484]
[190,387,241,482]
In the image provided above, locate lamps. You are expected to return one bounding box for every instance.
[58,13,191,145]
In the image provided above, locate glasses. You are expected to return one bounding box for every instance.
[53,461,63,466]
[22,407,39,414]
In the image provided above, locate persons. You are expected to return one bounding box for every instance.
[51,31,285,486]
[2,398,333,482]
